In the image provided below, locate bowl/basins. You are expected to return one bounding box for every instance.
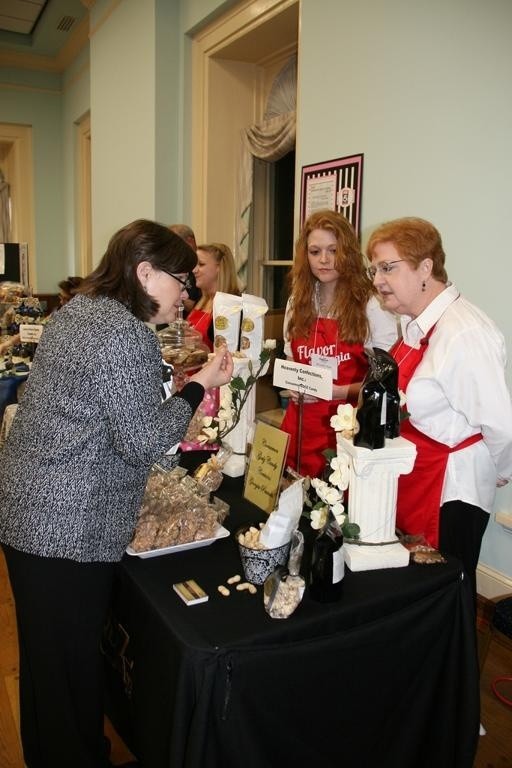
[236,526,292,585]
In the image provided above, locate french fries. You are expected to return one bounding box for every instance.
[249,585,257,594]
[237,524,265,550]
[236,583,250,591]
[218,585,230,596]
[227,574,240,584]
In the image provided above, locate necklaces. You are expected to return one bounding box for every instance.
[391,338,419,366]
[316,288,339,317]
[186,302,216,327]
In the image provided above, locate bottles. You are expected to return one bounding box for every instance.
[154,303,211,369]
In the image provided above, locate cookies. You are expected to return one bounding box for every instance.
[159,346,206,367]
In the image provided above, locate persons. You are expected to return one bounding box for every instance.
[0,277,84,356]
[281,210,399,502]
[172,242,240,476]
[0,217,234,768]
[171,220,192,318]
[367,218,511,612]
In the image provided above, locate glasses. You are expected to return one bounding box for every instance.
[160,266,193,291]
[368,257,405,278]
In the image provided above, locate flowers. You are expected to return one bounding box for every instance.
[195,336,277,458]
[305,387,413,544]
